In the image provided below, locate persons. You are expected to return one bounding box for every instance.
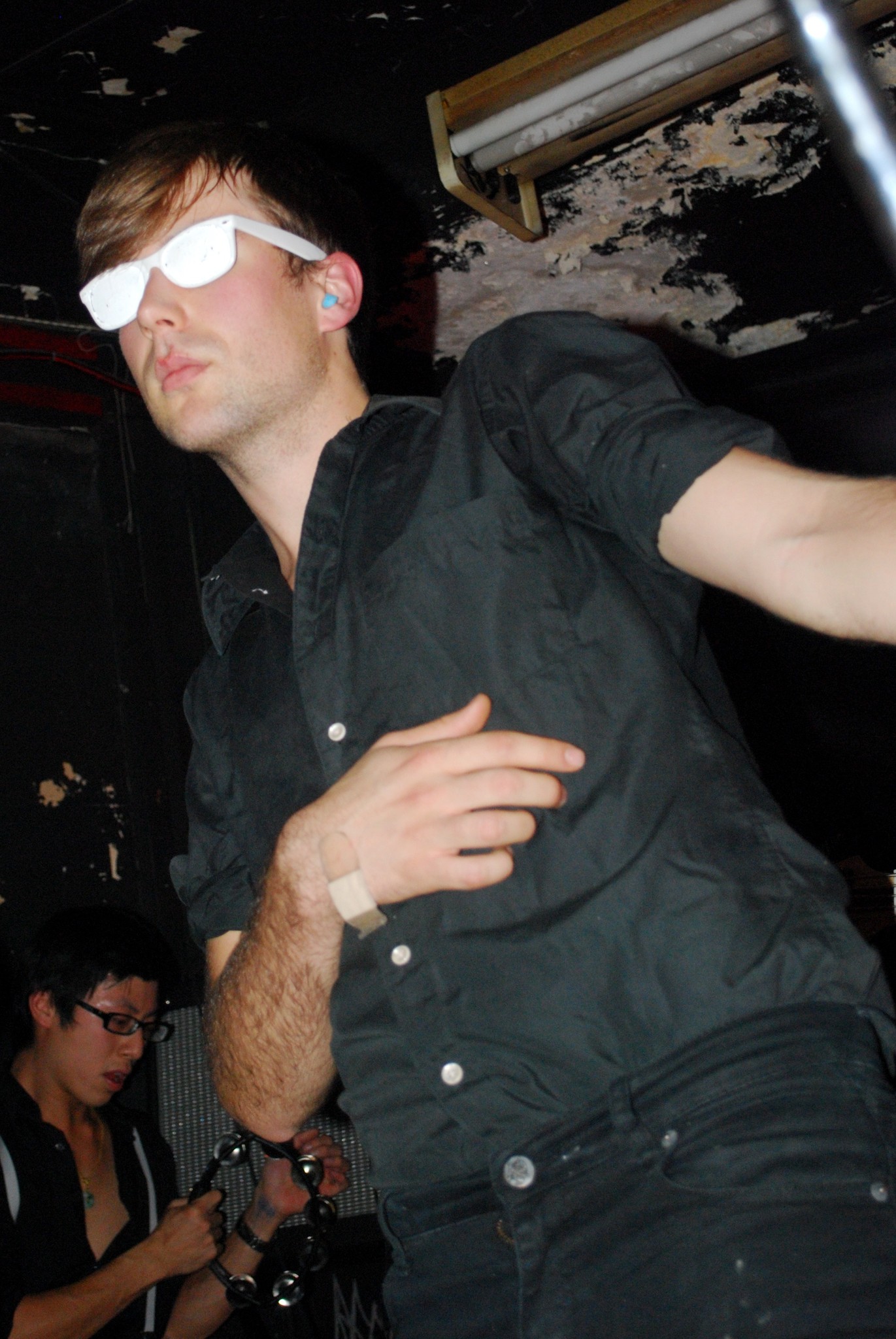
[0,914,352,1339]
[74,116,895,1338]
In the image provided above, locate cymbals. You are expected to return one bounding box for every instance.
[187,1128,341,1313]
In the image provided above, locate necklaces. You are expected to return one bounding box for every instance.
[78,1106,105,1208]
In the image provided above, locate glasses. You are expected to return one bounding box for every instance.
[78,215,326,331]
[74,996,172,1044]
